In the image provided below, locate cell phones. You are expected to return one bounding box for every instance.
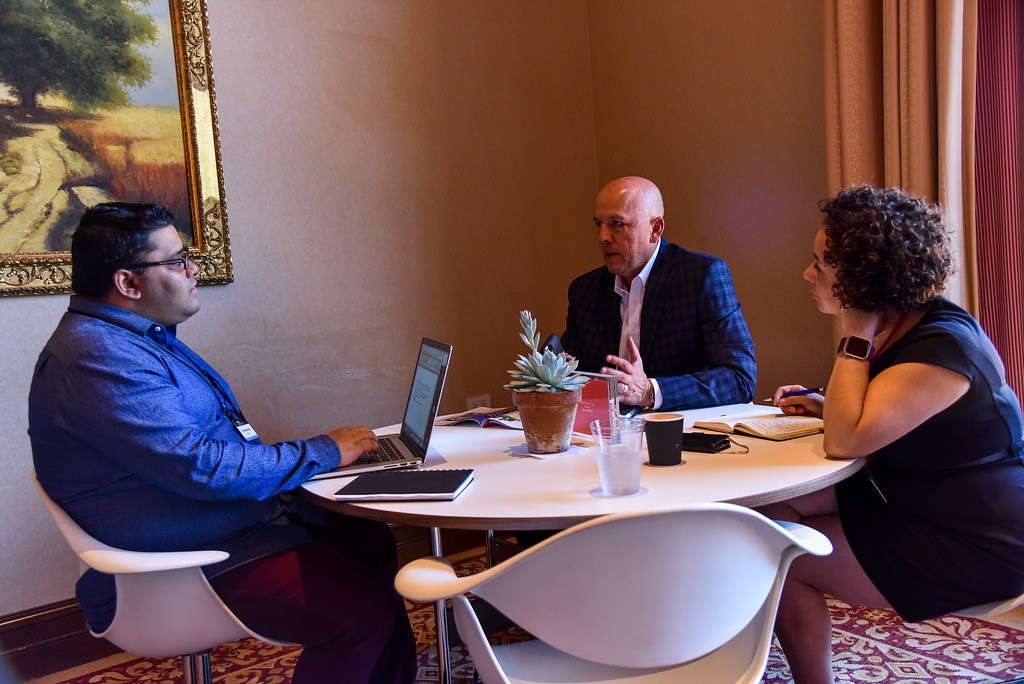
[541,334,564,356]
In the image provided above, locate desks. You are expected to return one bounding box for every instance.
[300,410,867,684]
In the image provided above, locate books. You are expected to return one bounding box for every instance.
[437,404,526,431]
[334,465,475,504]
[692,411,826,442]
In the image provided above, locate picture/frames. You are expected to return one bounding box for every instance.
[0,1,236,298]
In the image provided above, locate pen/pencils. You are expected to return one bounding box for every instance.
[764,386,826,402]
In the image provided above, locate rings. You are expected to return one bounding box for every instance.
[623,384,628,395]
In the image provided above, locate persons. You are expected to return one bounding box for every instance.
[542,176,760,412]
[28,199,420,684]
[752,181,1024,684]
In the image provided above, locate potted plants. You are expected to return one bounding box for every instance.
[502,310,590,454]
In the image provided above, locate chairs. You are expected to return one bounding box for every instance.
[394,502,833,684]
[31,471,302,684]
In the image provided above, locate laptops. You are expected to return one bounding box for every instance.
[309,336,453,482]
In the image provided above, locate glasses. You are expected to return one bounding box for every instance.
[111,247,189,289]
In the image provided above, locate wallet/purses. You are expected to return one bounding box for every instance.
[681,432,731,453]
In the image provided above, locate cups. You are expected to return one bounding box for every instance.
[642,413,685,465]
[589,417,647,496]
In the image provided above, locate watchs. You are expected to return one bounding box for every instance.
[836,332,878,365]
[648,383,655,408]
